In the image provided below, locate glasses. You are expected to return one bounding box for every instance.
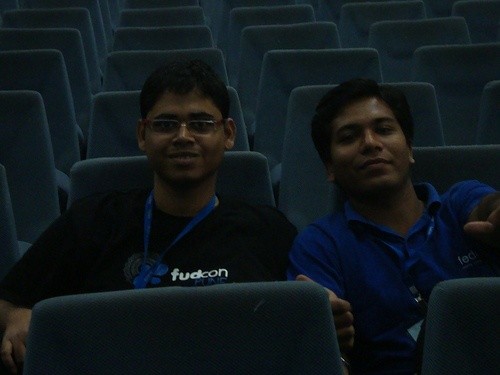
[144,117,223,135]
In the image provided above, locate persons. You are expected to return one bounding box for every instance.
[0,58,299,375]
[284,79,500,375]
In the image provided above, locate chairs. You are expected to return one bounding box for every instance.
[0,0,500,375]
